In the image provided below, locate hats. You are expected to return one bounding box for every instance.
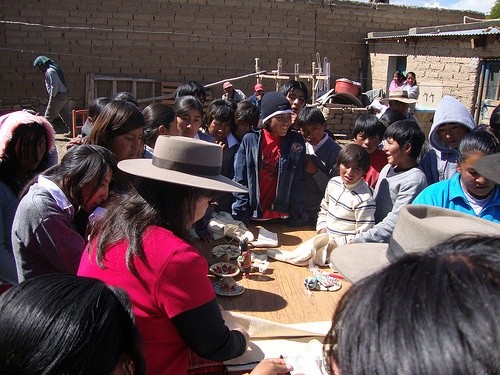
[223,82,232,89]
[255,84,263,91]
[379,90,417,105]
[261,91,294,123]
[117,135,252,193]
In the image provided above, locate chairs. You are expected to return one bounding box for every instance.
[71,109,89,137]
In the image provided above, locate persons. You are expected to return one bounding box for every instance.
[0,275,294,375]
[0,110,58,282]
[330,202,500,285]
[33,56,78,138]
[387,71,404,110]
[321,231,500,375]
[10,145,119,283]
[398,71,420,116]
[65,80,500,253]
[74,136,248,375]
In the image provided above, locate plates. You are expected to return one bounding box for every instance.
[214,282,245,296]
[209,244,241,276]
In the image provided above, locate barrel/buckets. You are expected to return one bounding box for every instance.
[335,81,352,94]
[351,83,362,97]
